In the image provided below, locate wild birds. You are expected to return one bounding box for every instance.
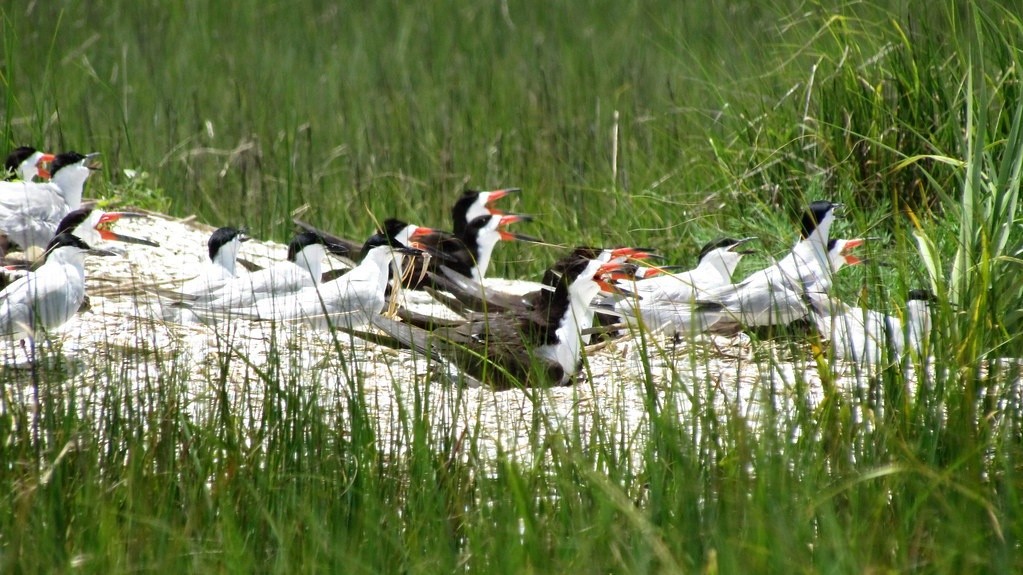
[0,145,161,375]
[137,187,959,392]
